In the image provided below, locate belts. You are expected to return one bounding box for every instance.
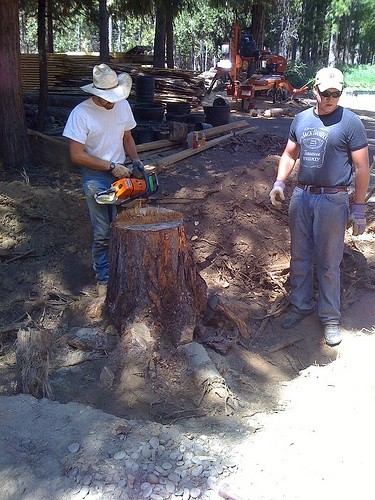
[296,182,347,194]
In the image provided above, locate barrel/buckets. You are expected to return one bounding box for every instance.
[194,121,213,130]
[131,103,166,123]
[165,101,192,124]
[203,105,230,127]
[136,75,155,102]
[213,97,229,105]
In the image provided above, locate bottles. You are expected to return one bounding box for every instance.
[202,132,206,145]
[193,131,199,149]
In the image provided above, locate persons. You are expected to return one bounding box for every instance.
[268,67,371,347]
[61,61,148,299]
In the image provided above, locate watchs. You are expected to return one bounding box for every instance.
[106,162,115,174]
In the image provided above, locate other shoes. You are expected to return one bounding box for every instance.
[322,324,341,345]
[281,310,308,329]
[96,282,107,298]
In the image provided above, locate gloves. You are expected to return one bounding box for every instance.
[111,163,133,179]
[132,160,144,177]
[269,180,286,208]
[346,203,367,236]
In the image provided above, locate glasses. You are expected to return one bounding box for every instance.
[317,87,342,98]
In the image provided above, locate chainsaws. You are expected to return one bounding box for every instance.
[94,173,207,208]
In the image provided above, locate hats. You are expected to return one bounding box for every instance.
[315,67,344,92]
[79,63,132,103]
